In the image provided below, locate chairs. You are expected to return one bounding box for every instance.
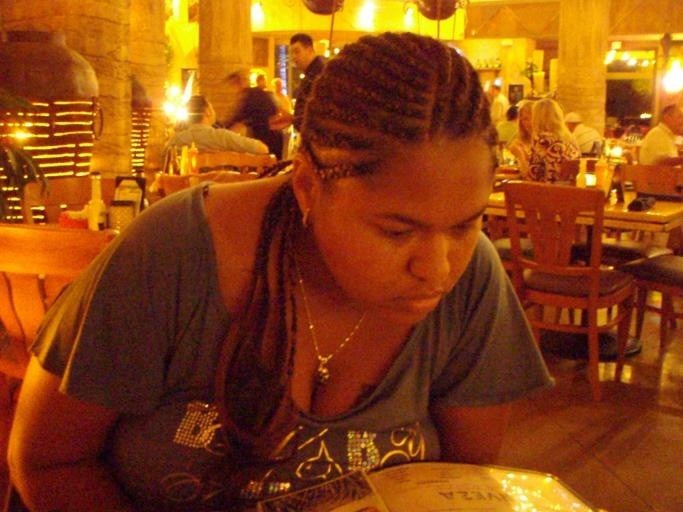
[484,139,682,404]
[0,139,293,410]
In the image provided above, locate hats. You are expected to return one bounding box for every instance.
[563,111,584,123]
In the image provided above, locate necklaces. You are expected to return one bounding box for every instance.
[286,242,369,388]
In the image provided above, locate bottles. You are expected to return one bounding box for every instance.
[88,172,106,231]
[595,156,610,190]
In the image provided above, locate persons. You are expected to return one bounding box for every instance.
[3,30,551,512]
[487,80,606,184]
[216,74,281,160]
[638,103,683,169]
[158,94,270,172]
[265,33,326,133]
